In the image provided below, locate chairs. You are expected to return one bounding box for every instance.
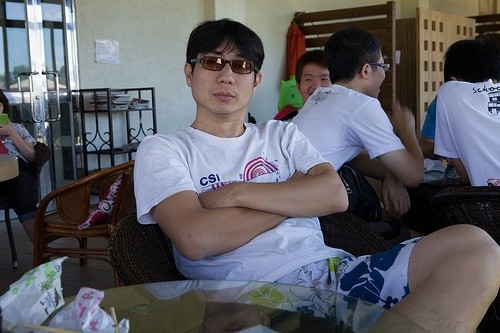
[109,210,190,286]
[339,165,379,222]
[431,186,500,246]
[32,161,136,289]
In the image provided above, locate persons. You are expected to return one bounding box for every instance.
[133,19,500,333]
[143,280,428,333]
[287,29,427,256]
[0,88,50,258]
[416,38,500,190]
[271,49,333,125]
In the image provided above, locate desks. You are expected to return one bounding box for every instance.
[39,279,430,333]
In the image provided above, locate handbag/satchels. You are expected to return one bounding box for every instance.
[338,162,382,222]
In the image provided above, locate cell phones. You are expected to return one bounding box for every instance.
[0,114,8,125]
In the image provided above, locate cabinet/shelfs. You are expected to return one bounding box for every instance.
[79,86,157,178]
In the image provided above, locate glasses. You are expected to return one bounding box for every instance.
[190,56,259,74]
[358,62,390,76]
